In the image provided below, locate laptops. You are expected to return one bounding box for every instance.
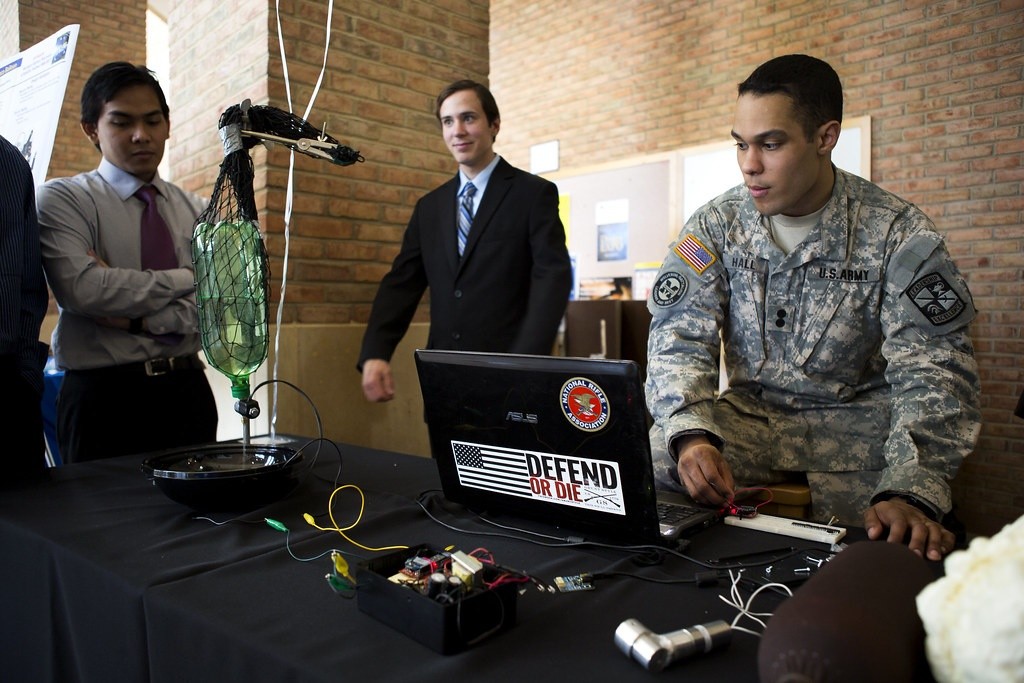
[413,349,728,549]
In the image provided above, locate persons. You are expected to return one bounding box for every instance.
[0,135,47,498]
[357,79,572,425]
[32,62,219,467]
[643,54,983,561]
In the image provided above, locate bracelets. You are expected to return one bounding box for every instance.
[129,316,143,334]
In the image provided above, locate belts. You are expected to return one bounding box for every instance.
[72,354,198,377]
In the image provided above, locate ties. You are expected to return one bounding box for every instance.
[457,182,478,257]
[132,185,186,346]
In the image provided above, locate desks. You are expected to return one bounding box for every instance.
[1,434,947,683]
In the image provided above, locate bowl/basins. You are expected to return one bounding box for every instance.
[140,444,304,514]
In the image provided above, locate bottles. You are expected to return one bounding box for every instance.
[192,221,269,398]
